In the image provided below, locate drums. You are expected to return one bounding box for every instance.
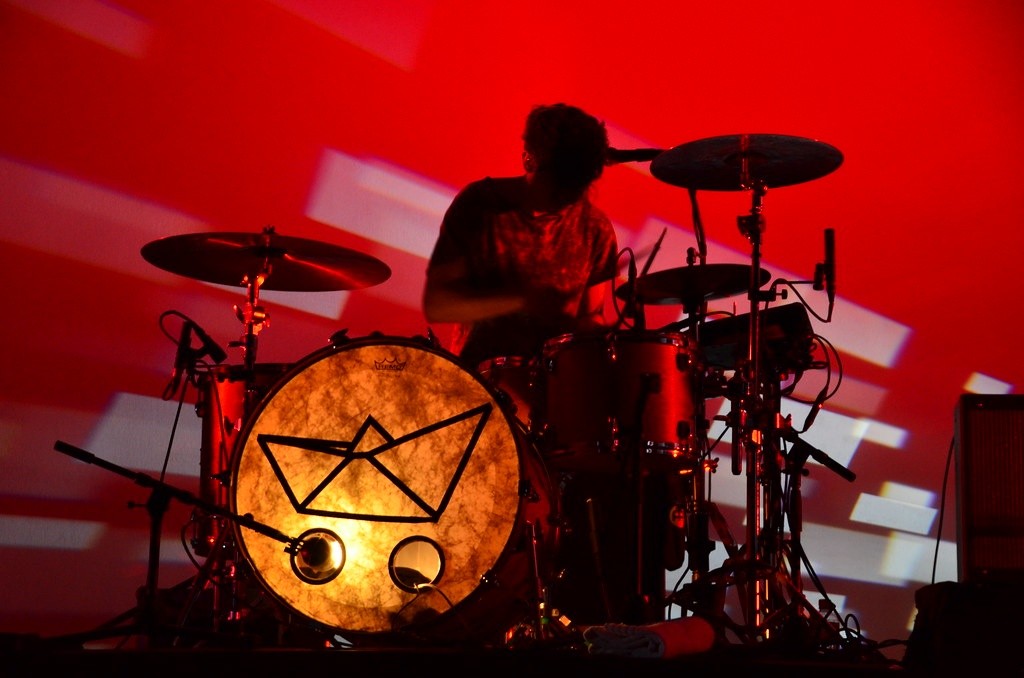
[222,327,562,644]
[477,352,545,452]
[699,303,815,370]
[193,362,291,558]
[540,327,705,483]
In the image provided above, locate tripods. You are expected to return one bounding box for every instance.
[55,269,303,649]
[626,178,858,658]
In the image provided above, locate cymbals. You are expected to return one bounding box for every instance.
[139,232,393,293]
[617,263,772,306]
[650,133,844,192]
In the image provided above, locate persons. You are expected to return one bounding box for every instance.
[423,104,617,358]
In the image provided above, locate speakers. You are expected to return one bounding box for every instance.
[954,392,1024,588]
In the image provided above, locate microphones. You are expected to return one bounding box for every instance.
[628,252,640,317]
[183,316,227,364]
[298,537,330,566]
[824,230,836,304]
